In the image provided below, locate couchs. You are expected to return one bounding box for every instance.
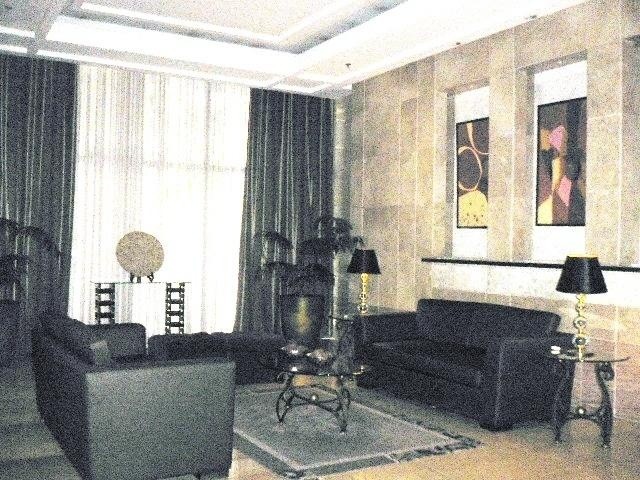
[31,324,237,480]
[353,311,578,432]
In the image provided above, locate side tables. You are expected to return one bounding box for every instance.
[328,301,416,357]
[539,347,630,449]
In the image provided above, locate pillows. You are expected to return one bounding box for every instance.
[474,302,562,351]
[39,309,111,366]
[416,298,476,349]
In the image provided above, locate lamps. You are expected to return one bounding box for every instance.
[347,247,382,312]
[555,255,609,361]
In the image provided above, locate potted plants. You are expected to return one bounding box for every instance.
[0,216,64,368]
[252,214,368,350]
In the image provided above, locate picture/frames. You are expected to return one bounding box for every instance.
[456,117,489,228]
[535,97,587,225]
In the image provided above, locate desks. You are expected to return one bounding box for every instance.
[89,277,192,336]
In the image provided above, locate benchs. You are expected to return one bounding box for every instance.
[148,331,287,383]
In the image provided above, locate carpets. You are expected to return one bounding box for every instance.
[232,378,485,480]
